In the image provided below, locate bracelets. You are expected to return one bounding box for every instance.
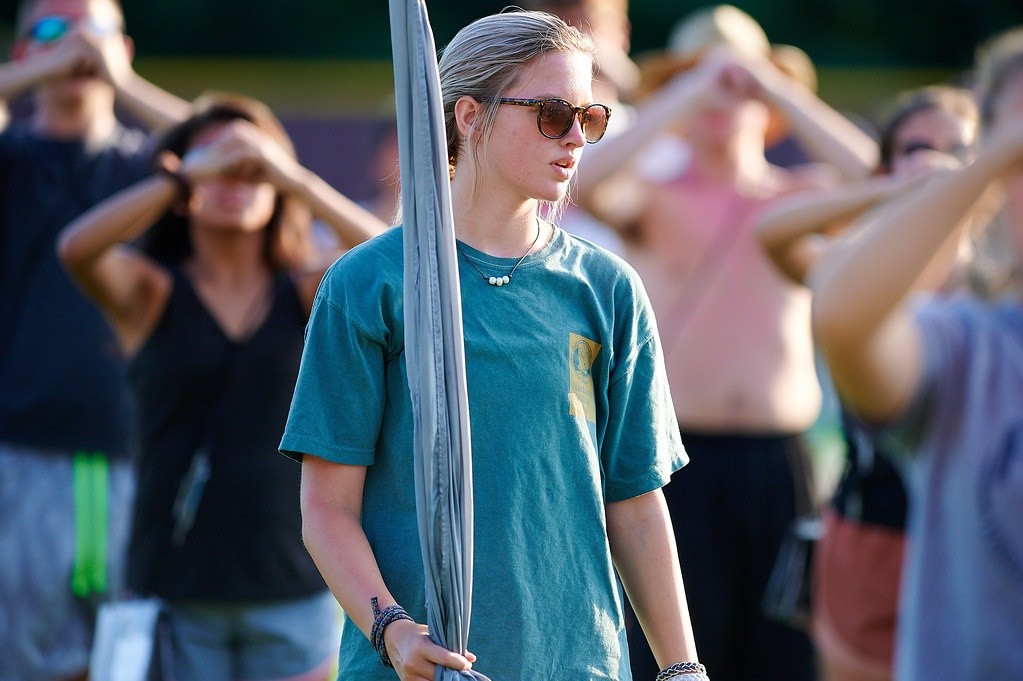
[370,596,415,668]
[155,161,192,218]
[656,662,710,681]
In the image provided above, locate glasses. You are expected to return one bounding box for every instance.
[22,16,68,45]
[473,97,612,144]
[899,141,974,165]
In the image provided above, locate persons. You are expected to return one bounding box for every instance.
[0,1,189,681]
[810,52,1022,681]
[571,0,879,681]
[533,0,645,258]
[751,86,986,681]
[280,10,711,681]
[59,93,394,681]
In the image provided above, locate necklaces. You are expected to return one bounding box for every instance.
[456,217,540,286]
